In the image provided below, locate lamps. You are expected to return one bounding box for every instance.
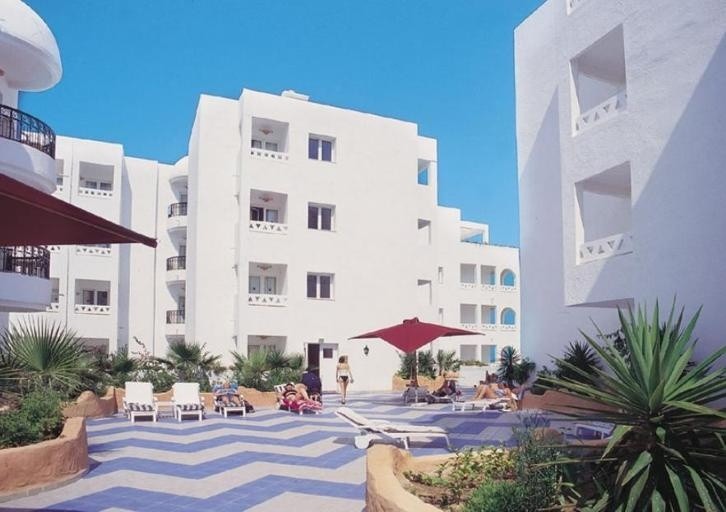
[364,346,370,355]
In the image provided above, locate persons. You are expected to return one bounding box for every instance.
[500,384,518,412]
[334,355,354,405]
[440,379,456,395]
[299,366,323,403]
[212,380,243,408]
[471,382,520,402]
[277,383,322,408]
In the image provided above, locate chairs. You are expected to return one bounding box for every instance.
[212,383,253,418]
[274,382,320,415]
[334,381,524,451]
[574,421,616,440]
[122,381,159,424]
[170,382,207,422]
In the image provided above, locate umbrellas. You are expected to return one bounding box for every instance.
[344,316,486,384]
[0,171,157,252]
